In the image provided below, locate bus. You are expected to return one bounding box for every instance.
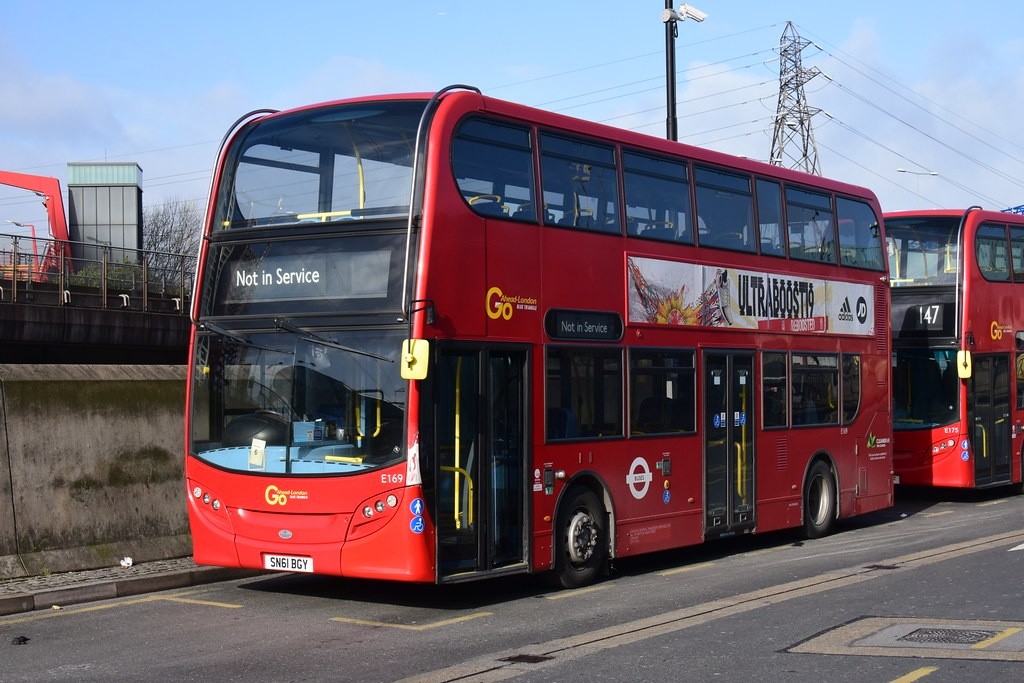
[819,205,1024,503]
[179,84,900,588]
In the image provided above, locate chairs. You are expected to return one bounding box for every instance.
[944,266,960,274]
[638,396,675,434]
[469,193,855,264]
[550,406,580,441]
[980,266,1024,273]
